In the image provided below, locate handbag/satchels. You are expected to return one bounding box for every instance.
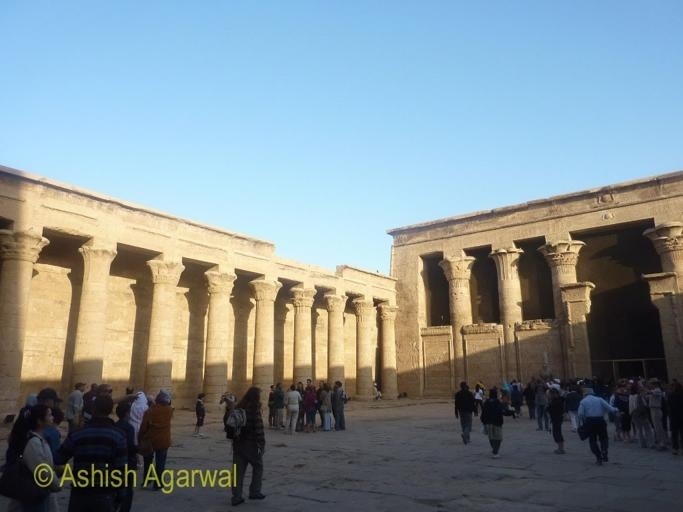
[224,404,247,439]
[576,414,589,441]
[1,434,51,509]
[630,394,650,420]
[481,422,488,435]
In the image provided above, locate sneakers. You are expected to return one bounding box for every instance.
[231,491,266,504]
[267,421,345,434]
[192,432,204,437]
[554,448,568,455]
[141,478,165,490]
[488,451,500,458]
[613,431,671,452]
[460,431,472,445]
[534,426,551,431]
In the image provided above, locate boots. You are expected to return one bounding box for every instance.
[594,451,610,464]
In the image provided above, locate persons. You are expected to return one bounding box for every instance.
[219,390,234,432]
[226,386,265,506]
[267,378,345,432]
[193,392,205,436]
[0,381,173,511]
[453,372,683,464]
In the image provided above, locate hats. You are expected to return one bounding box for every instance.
[36,387,64,403]
